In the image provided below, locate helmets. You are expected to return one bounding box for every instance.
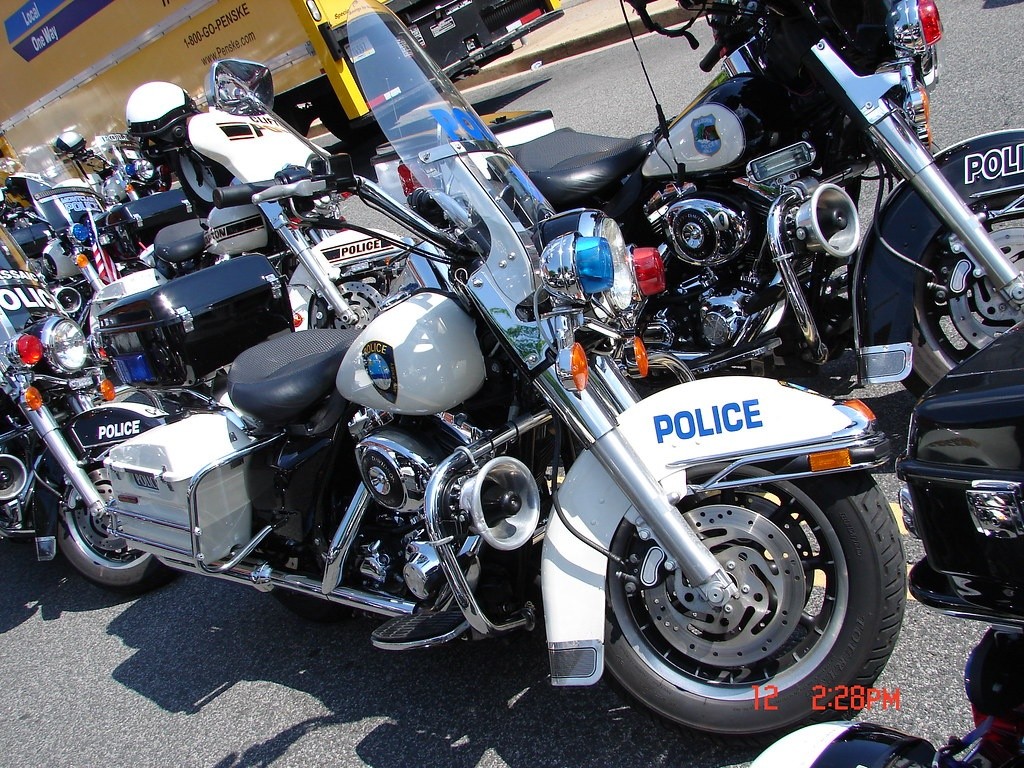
[128,80,196,138]
[55,130,88,155]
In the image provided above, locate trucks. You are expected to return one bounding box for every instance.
[2,1,564,154]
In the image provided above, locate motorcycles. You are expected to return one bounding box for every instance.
[1,1,1023,768]
